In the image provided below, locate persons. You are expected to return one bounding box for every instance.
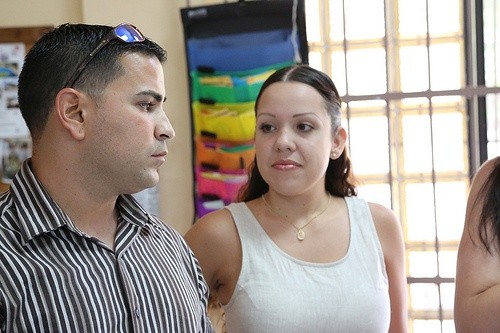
[454,156,500,333]
[183,65,408,333]
[0,24,214,333]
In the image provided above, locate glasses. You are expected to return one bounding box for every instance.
[64,23,145,88]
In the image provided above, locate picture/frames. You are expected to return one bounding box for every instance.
[0,26,54,196]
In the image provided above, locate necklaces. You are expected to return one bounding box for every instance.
[261,191,331,240]
